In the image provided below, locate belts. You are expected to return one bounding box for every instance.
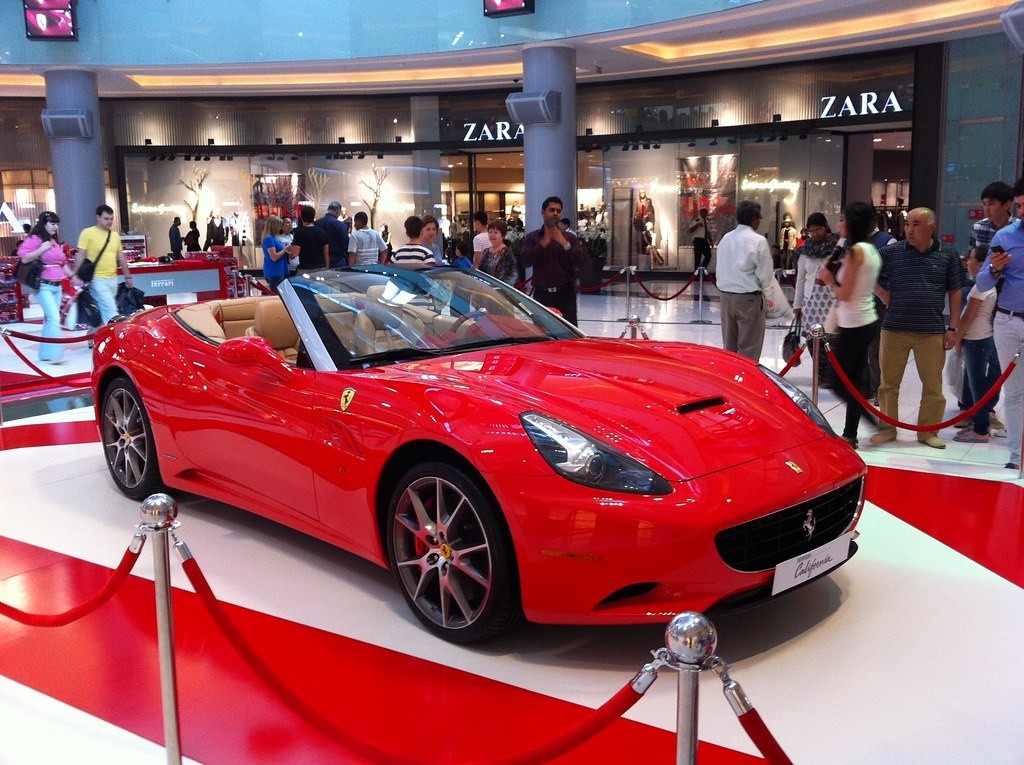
[41,279,60,286]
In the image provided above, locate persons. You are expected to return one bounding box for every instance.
[716,201,794,363]
[518,197,584,329]
[73,204,133,327]
[783,180,1024,472]
[261,201,392,292]
[391,215,443,316]
[169,217,202,259]
[687,208,714,276]
[203,208,252,270]
[578,202,610,264]
[779,212,797,270]
[634,189,665,270]
[11,211,83,364]
[449,206,522,319]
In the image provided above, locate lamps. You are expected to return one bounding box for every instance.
[395,136,402,143]
[326,151,355,160]
[727,136,737,144]
[755,133,764,143]
[772,114,781,122]
[799,131,808,140]
[585,124,695,153]
[357,151,365,160]
[711,119,719,127]
[145,138,152,146]
[767,131,778,142]
[824,133,833,143]
[275,138,283,145]
[779,132,788,141]
[339,137,345,144]
[149,153,235,161]
[266,152,299,160]
[207,138,215,145]
[377,153,383,159]
[708,137,718,146]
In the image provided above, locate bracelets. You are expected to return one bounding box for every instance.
[947,327,959,333]
[989,262,1001,277]
[285,250,293,254]
[68,273,76,280]
[124,276,133,280]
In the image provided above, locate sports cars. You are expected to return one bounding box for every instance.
[88,255,871,645]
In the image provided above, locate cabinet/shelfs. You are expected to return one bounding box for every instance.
[0,256,23,323]
[117,235,147,268]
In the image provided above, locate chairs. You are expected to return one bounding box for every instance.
[352,284,425,358]
[245,299,299,364]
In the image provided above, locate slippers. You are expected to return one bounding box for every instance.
[953,426,991,443]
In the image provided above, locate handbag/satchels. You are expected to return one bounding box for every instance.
[782,312,801,367]
[11,259,42,290]
[76,258,95,282]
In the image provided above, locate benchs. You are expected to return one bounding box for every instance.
[216,291,366,353]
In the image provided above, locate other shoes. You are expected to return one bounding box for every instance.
[841,436,858,450]
[869,430,897,444]
[49,358,69,364]
[954,418,974,428]
[922,436,946,449]
[994,429,1007,437]
[990,414,1004,429]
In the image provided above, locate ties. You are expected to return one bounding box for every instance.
[704,218,707,239]
